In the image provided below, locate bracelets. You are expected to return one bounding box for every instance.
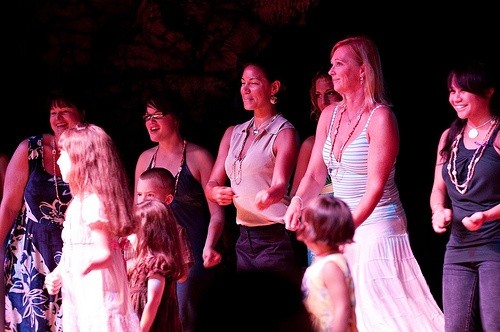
[290,196,304,211]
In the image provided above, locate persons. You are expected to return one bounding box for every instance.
[1,37,500,332]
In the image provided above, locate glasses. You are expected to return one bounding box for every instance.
[142,112,170,121]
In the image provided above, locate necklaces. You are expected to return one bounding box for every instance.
[453,119,496,195]
[53,136,73,205]
[234,113,279,186]
[344,102,364,126]
[327,103,369,179]
[447,118,500,188]
[253,112,281,136]
[150,139,187,197]
[465,114,496,139]
